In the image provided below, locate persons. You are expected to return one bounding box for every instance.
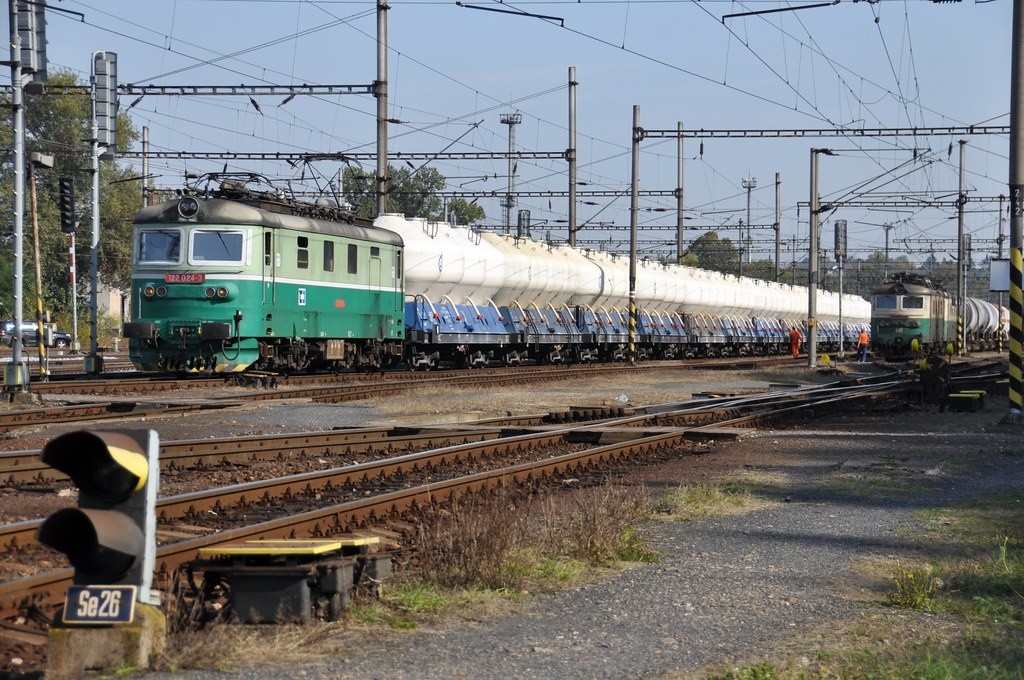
[856,329,869,363]
[788,326,802,359]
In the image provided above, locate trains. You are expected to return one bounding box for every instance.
[872,283,1010,362]
[123,197,872,378]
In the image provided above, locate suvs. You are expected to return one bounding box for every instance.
[0,320,71,350]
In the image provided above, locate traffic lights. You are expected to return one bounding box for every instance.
[33,428,161,606]
[59,178,75,233]
